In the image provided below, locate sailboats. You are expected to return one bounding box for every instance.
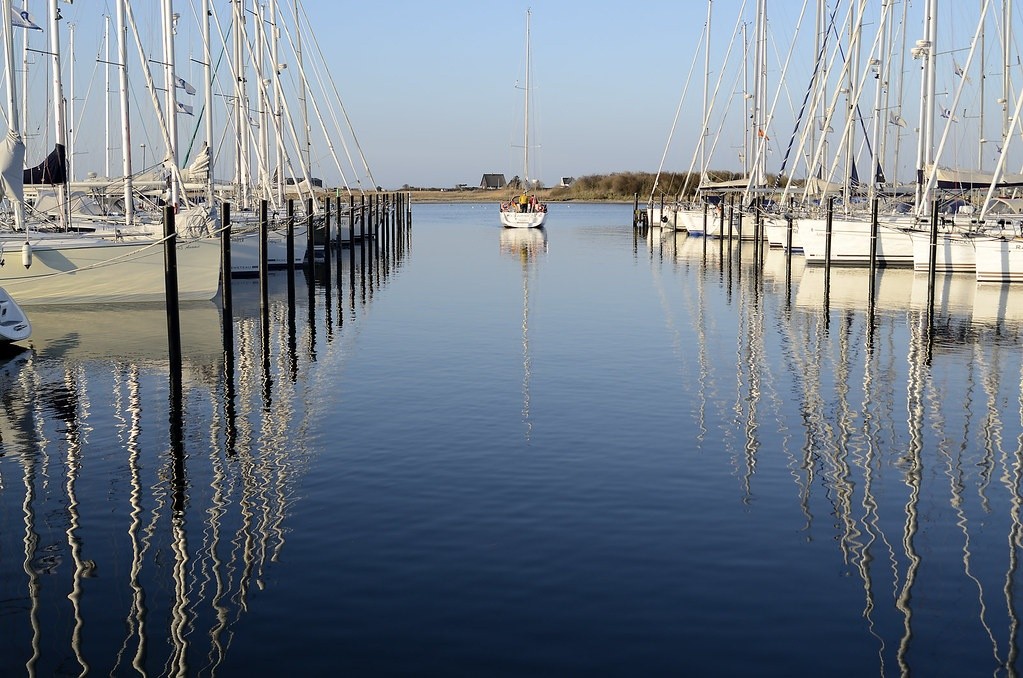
[645,0,1023,285]
[0,0,392,346]
[498,7,551,229]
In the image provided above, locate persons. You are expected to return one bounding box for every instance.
[529,194,539,213]
[518,191,528,213]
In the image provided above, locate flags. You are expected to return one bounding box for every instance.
[175,102,194,116]
[173,73,196,95]
[11,5,43,32]
[939,104,959,123]
[758,127,769,141]
[818,120,834,133]
[249,115,260,126]
[953,58,973,85]
[889,110,907,127]
